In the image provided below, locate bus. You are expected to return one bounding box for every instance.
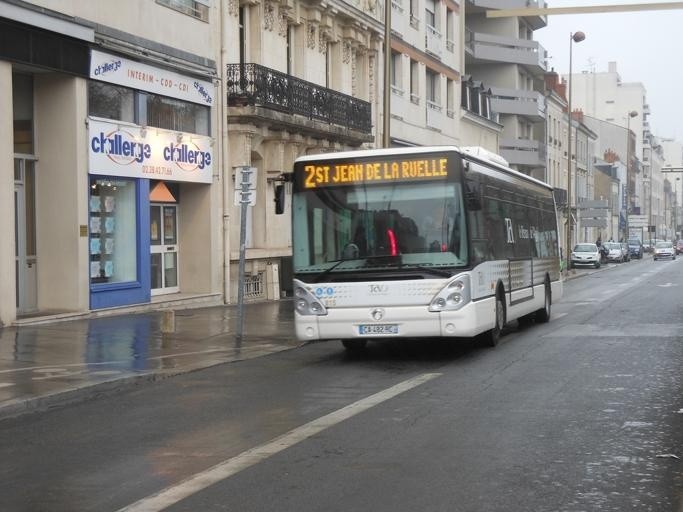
[273,145,560,348]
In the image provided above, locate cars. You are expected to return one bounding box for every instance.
[571,239,683,268]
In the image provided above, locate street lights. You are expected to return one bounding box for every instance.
[625,110,638,241]
[567,31,585,270]
[674,177,680,235]
